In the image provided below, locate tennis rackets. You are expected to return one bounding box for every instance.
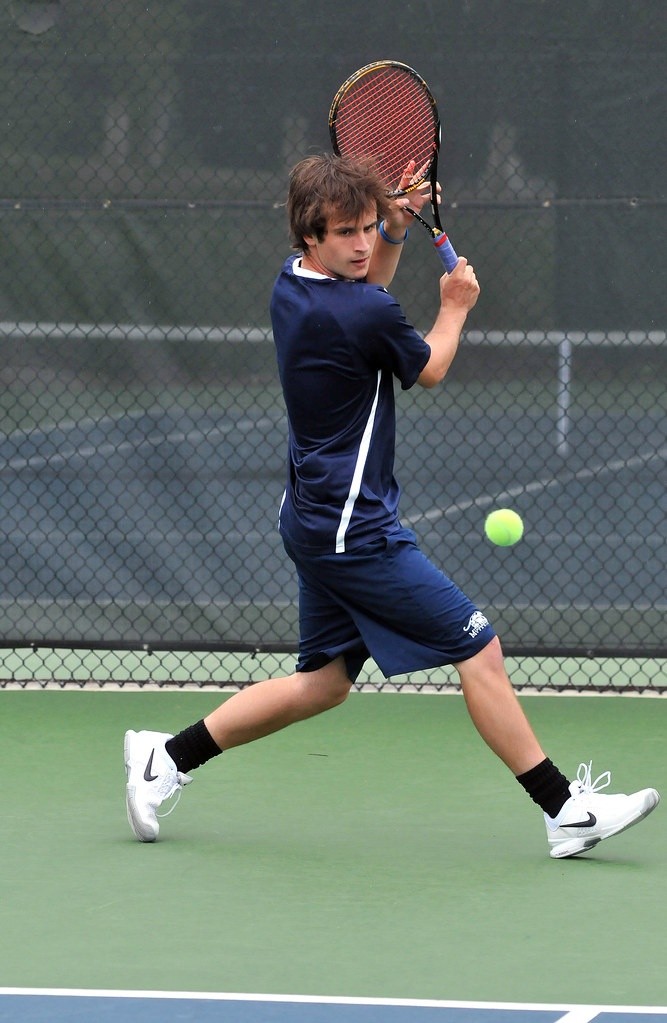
[328,58,460,274]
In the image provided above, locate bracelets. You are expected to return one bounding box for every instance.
[377,219,410,246]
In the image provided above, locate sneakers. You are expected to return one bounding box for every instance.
[543,780,658,858]
[124,729,192,842]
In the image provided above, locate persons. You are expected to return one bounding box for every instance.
[117,152,666,862]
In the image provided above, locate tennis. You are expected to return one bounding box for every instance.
[484,508,524,547]
